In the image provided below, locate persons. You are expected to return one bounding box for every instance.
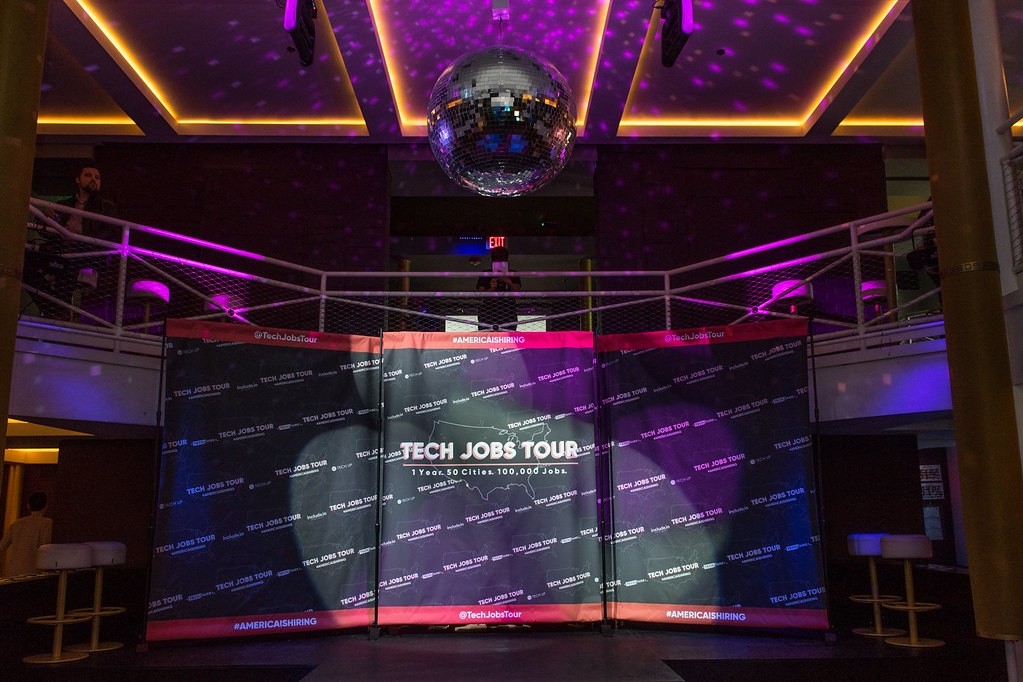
[35,166,131,307]
[907,195,943,304]
[476,246,522,331]
[0,492,53,579]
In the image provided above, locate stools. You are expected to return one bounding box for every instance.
[204,293,244,323]
[847,532,945,647]
[128,278,171,333]
[22,541,127,664]
[68,267,98,323]
[862,280,888,326]
[772,279,814,315]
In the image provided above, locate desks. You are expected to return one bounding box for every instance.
[858,217,914,325]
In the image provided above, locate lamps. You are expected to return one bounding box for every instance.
[427,0,578,197]
